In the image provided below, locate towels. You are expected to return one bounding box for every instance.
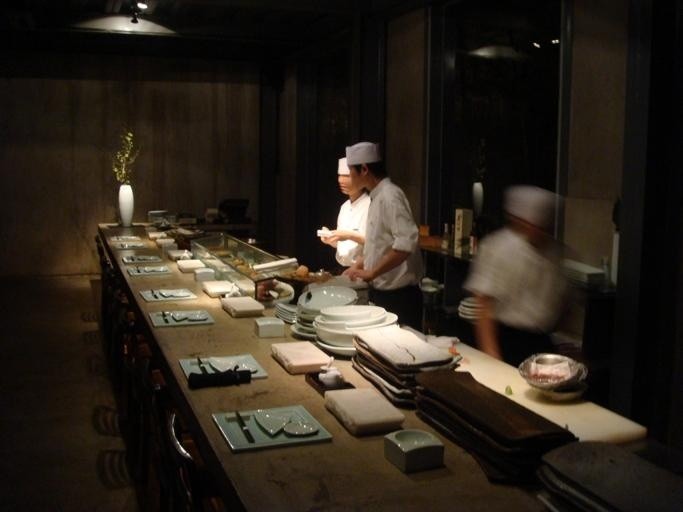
[186,369,252,389]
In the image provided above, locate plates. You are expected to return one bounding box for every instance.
[519,353,589,402]
[457,297,484,319]
[212,404,333,452]
[179,355,268,378]
[122,255,168,275]
[275,286,444,404]
[149,310,214,328]
[140,288,196,301]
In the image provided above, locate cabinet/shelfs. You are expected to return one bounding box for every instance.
[95,230,237,512]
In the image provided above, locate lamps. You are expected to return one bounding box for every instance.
[72,1,178,39]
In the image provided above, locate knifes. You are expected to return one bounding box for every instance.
[235,409,255,445]
[162,311,168,324]
[196,357,208,375]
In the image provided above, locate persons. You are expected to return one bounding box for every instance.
[321,142,425,333]
[465,186,563,371]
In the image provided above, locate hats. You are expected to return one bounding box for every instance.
[337,142,380,176]
[504,185,565,228]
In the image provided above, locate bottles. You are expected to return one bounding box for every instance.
[469,222,478,256]
[441,223,454,250]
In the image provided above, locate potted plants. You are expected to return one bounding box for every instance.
[110,130,143,226]
